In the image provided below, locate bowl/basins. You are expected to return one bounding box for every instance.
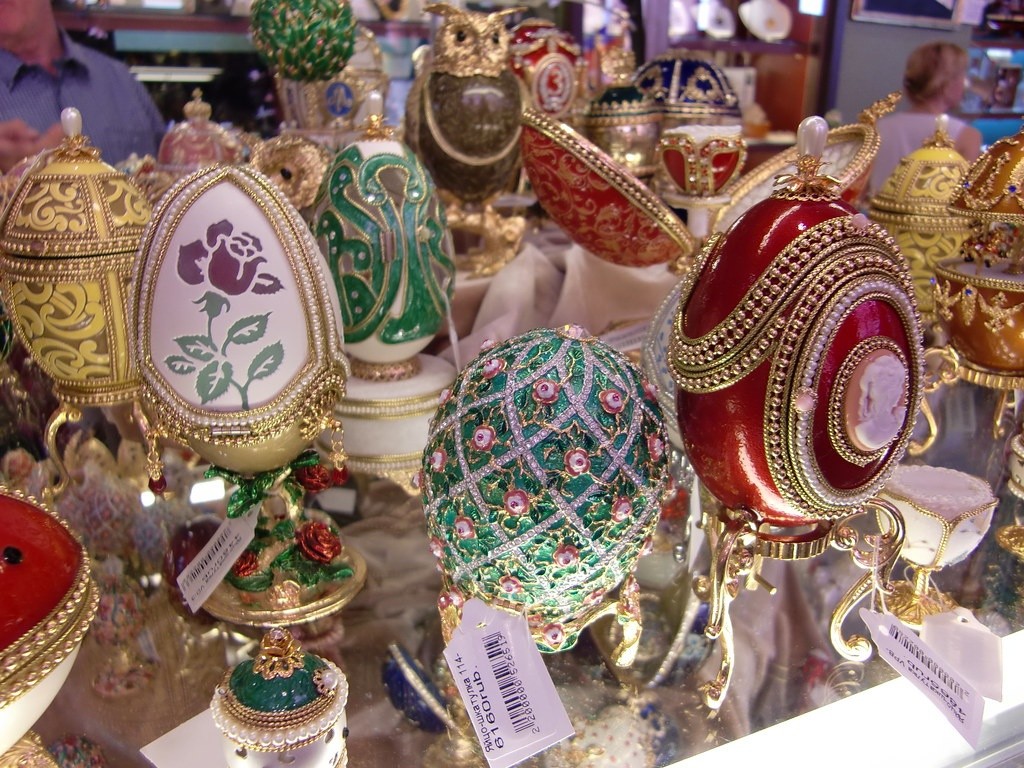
[660,124,741,196]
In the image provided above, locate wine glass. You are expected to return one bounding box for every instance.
[876,465,999,631]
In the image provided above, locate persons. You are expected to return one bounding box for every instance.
[0,0,163,175]
[993,68,1020,108]
[868,41,982,196]
